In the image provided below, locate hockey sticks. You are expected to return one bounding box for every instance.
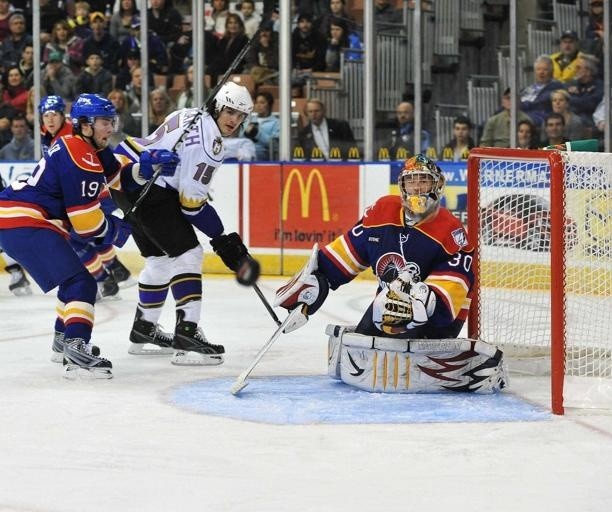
[231,303,304,396]
[123,1,276,221]
[253,281,308,333]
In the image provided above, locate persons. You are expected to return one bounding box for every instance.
[0,0,612,162]
[113,81,254,354]
[0,175,28,289]
[272,153,474,339]
[0,92,181,368]
[38,94,132,299]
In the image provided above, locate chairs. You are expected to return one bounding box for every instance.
[343,0,430,28]
[150,72,341,127]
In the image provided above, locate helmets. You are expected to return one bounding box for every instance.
[70,92,120,134]
[397,153,446,214]
[208,81,255,133]
[38,95,66,116]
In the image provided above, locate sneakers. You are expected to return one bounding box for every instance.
[62,337,112,369]
[130,307,174,347]
[5,263,30,291]
[173,309,224,354]
[97,266,119,297]
[107,255,130,283]
[52,331,65,353]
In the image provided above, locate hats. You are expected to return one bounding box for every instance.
[90,11,104,22]
[48,51,63,63]
[559,29,578,40]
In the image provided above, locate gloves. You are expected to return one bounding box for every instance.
[209,232,261,286]
[138,148,180,180]
[100,213,132,249]
